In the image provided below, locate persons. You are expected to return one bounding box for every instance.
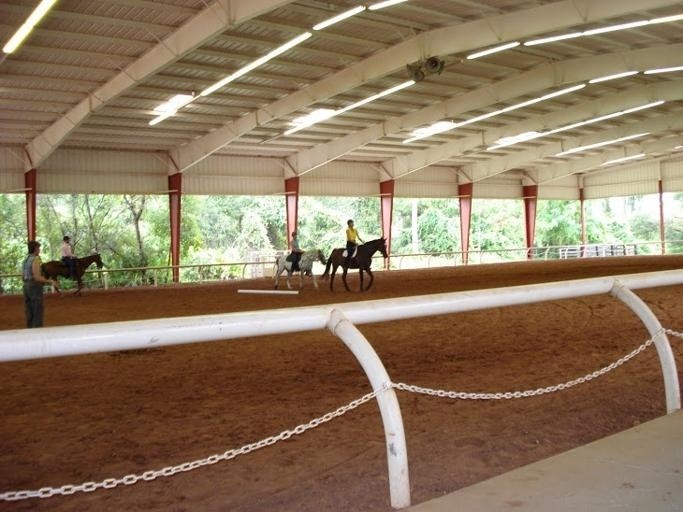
[345,219,366,265]
[290,231,302,272]
[19,240,56,327]
[61,235,77,279]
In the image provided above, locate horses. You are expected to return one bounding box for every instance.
[272,247,327,295]
[40,253,103,297]
[320,235,388,292]
